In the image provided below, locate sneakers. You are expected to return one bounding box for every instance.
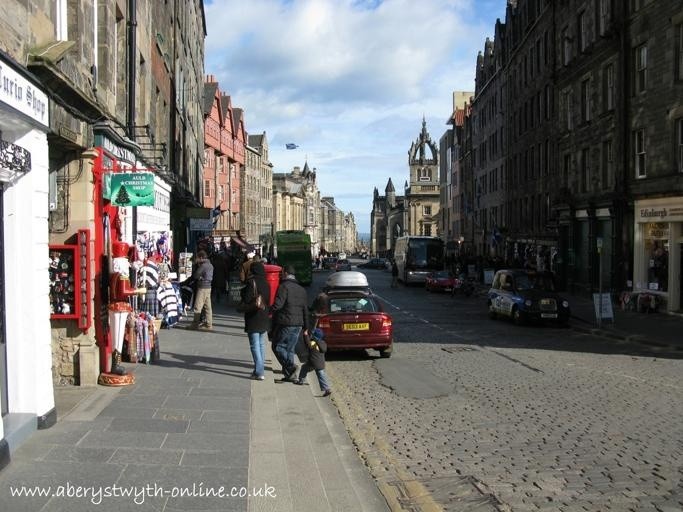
[251,371,264,380]
[324,389,331,396]
[282,365,303,385]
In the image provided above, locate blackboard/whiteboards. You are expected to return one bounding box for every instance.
[48,244,81,321]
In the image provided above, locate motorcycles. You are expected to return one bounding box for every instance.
[449,273,476,299]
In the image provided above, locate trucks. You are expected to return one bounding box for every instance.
[258,231,313,286]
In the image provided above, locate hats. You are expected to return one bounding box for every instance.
[309,328,323,341]
[250,262,265,276]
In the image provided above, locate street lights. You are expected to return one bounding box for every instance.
[460,233,466,276]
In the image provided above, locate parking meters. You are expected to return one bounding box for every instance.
[596,236,605,329]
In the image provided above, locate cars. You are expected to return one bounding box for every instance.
[322,250,369,271]
[487,269,569,325]
[309,270,393,359]
[425,271,457,292]
[358,258,386,270]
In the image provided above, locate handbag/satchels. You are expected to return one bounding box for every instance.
[236,295,265,313]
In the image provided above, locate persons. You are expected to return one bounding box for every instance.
[215,255,228,304]
[185,250,214,330]
[270,264,309,384]
[179,255,206,327]
[106,241,147,377]
[391,258,399,288]
[297,328,332,397]
[240,251,256,284]
[240,261,270,381]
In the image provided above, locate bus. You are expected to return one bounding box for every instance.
[392,235,444,286]
